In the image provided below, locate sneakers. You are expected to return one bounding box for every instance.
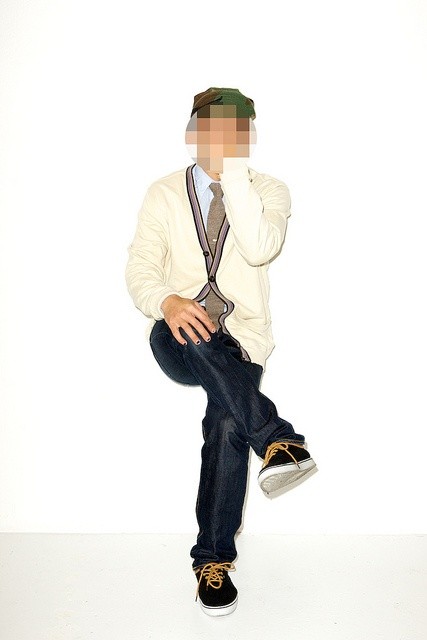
[257,444,316,495]
[195,563,238,615]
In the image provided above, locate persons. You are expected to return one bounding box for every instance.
[125,89,317,618]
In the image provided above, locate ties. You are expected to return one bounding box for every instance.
[208,183,224,331]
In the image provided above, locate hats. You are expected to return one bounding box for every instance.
[190,87,254,120]
